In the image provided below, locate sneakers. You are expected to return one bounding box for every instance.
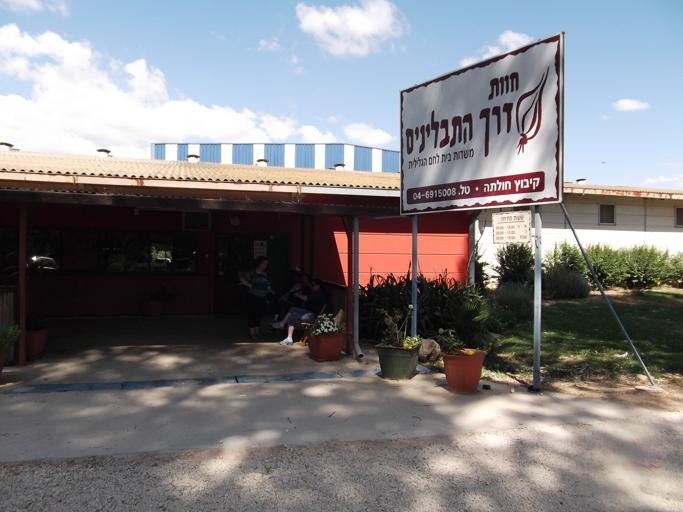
[280,338,293,345]
[272,322,284,329]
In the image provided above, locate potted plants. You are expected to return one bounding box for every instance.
[435,326,486,394]
[140,284,176,319]
[372,304,422,380]
[0,319,21,372]
[306,333,346,363]
[2,251,61,360]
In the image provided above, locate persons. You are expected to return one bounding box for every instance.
[274,277,327,346]
[238,256,274,340]
[267,273,311,327]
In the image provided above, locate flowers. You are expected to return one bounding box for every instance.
[310,312,346,335]
